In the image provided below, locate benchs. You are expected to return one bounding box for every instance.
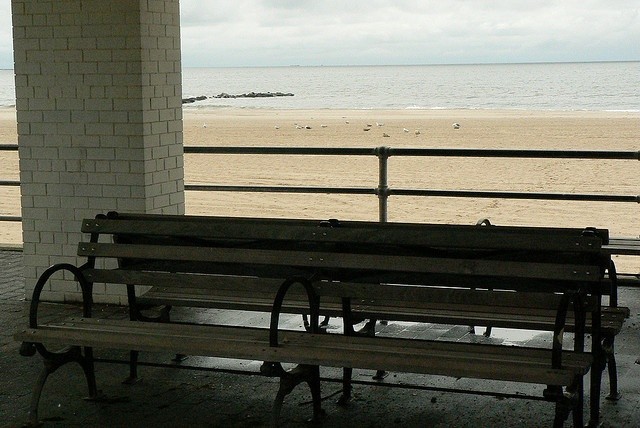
[105,206,630,428]
[12,209,603,428]
